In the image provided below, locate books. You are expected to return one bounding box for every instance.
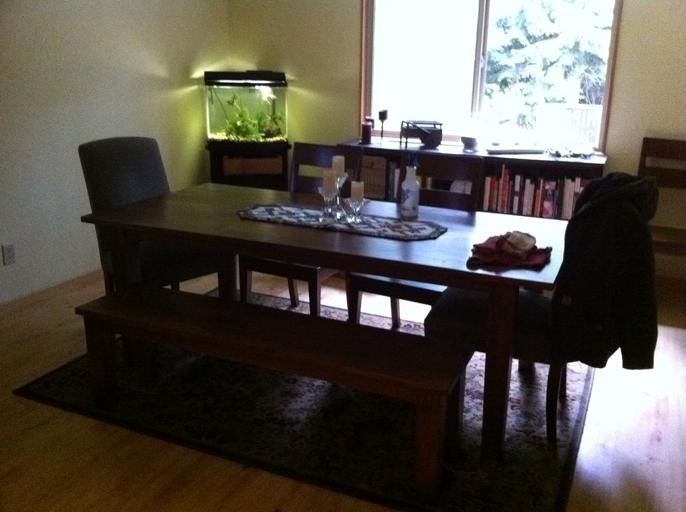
[482,164,588,220]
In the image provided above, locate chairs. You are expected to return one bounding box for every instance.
[76,137,658,444]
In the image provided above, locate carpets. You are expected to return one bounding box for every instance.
[13,285,596,512]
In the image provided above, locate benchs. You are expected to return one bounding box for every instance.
[74,287,465,502]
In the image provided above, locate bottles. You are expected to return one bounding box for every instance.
[399,160,421,221]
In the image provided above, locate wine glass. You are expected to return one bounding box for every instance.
[327,172,350,217]
[319,186,339,224]
[343,197,370,225]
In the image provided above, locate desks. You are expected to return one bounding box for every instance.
[79,183,574,470]
